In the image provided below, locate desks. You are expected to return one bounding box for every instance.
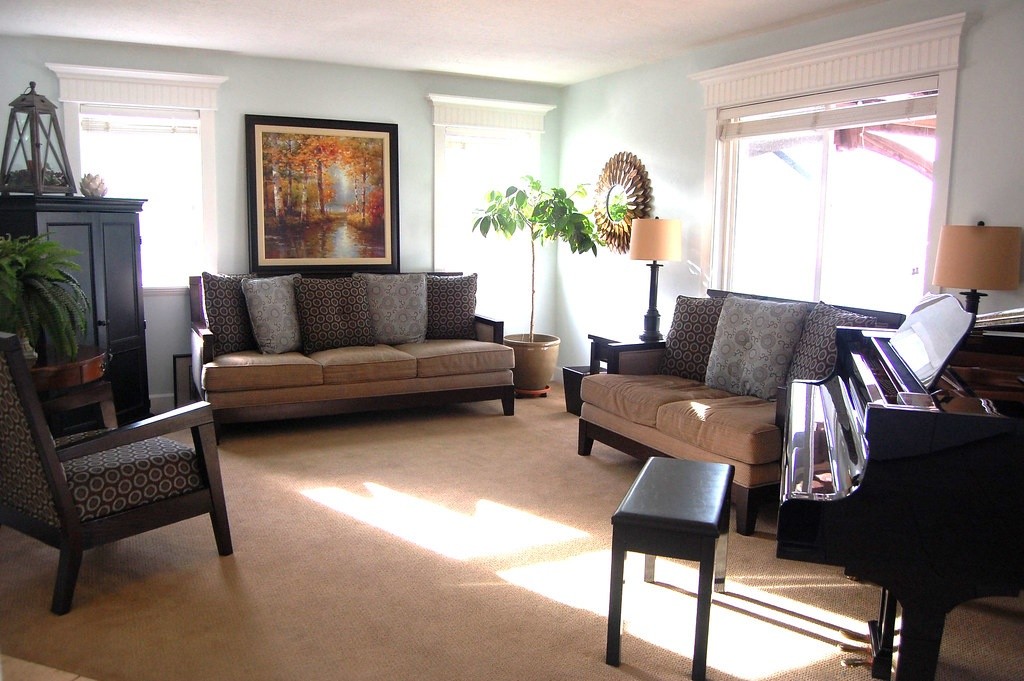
[32,344,108,439]
[588,332,667,376]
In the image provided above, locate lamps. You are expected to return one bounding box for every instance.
[628,217,683,343]
[932,219,1024,332]
[0,81,78,199]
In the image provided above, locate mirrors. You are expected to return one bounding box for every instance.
[591,150,656,256]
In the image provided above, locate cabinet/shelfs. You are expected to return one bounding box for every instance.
[0,193,155,451]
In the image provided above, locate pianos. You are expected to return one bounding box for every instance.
[776,289,1022,681]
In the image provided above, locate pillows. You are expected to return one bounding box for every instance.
[240,273,304,355]
[426,272,478,341]
[201,271,258,358]
[352,272,428,345]
[292,276,375,356]
[785,300,876,389]
[705,293,810,403]
[656,295,726,383]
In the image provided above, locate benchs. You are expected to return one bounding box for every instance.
[189,271,519,446]
[577,289,908,537]
[605,456,734,681]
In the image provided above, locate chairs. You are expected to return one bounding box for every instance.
[0,330,234,616]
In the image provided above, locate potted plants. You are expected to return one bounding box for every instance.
[473,174,608,401]
[0,231,94,368]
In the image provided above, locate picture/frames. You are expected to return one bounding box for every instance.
[244,113,401,279]
[172,353,206,409]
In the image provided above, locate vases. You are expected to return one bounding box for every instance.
[562,365,607,417]
